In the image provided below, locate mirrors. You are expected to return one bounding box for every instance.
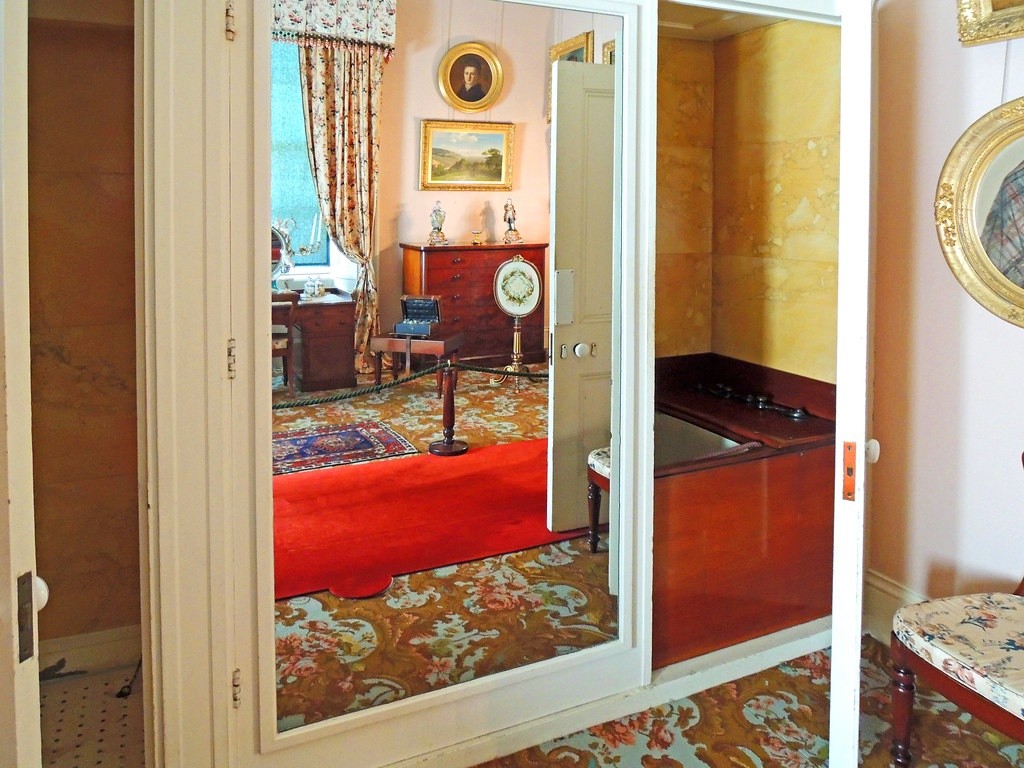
[253,0,653,752]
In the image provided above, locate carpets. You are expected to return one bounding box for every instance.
[273,419,419,476]
[272,435,608,605]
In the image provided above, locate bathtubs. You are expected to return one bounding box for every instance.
[651,382,837,670]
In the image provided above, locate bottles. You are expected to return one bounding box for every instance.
[315,276,325,296]
[304,277,315,296]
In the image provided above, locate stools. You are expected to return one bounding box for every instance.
[586,446,610,554]
[889,579,1024,768]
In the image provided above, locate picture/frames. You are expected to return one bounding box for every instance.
[934,94,1024,329]
[545,30,593,122]
[421,118,512,193]
[435,42,503,113]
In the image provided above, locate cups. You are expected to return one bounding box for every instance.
[472,231,483,244]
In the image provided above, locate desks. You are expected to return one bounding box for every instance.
[269,286,358,392]
[367,329,463,397]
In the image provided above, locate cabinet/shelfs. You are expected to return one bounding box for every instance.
[397,241,545,368]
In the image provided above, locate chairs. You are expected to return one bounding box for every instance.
[271,291,300,400]
